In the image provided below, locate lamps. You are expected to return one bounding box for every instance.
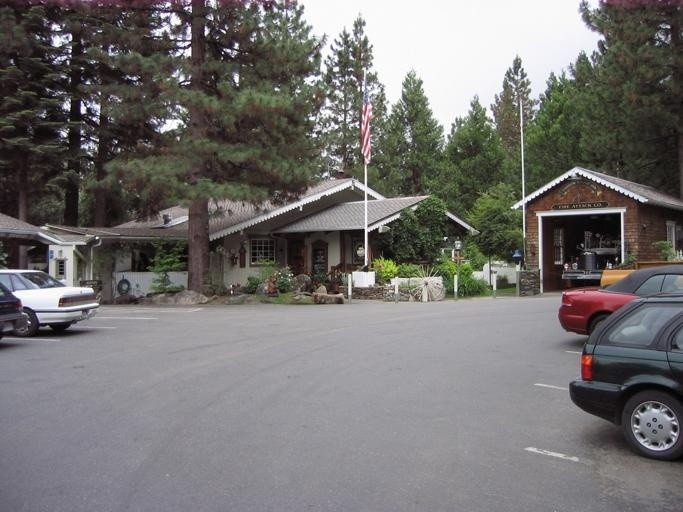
[511,247,522,258]
[229,253,239,265]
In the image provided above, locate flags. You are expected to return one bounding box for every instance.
[359,72,376,166]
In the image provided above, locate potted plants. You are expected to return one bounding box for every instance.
[333,274,346,291]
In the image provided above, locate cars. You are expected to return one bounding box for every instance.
[558,265,683,459]
[0,269,100,337]
